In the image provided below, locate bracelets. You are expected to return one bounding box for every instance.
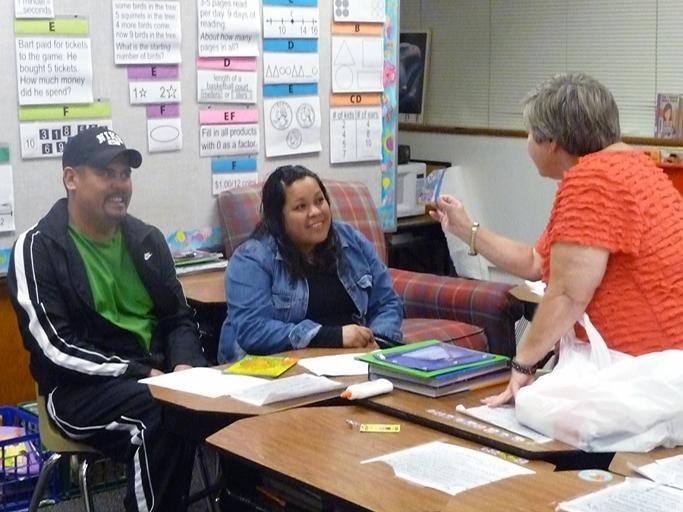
[466,219,481,258]
[509,356,538,377]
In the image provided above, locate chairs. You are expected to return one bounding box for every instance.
[215,180,524,356]
[27,382,109,511]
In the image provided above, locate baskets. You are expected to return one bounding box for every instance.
[17,399,129,500]
[0,405,61,512]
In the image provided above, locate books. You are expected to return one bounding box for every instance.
[350,337,513,400]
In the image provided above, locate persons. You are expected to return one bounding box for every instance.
[214,163,403,365]
[4,124,213,512]
[428,69,682,411]
[660,103,675,137]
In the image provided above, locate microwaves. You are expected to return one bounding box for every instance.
[397,162,427,219]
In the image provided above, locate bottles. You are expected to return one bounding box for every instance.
[339,377,396,402]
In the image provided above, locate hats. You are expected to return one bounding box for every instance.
[63,127,142,168]
